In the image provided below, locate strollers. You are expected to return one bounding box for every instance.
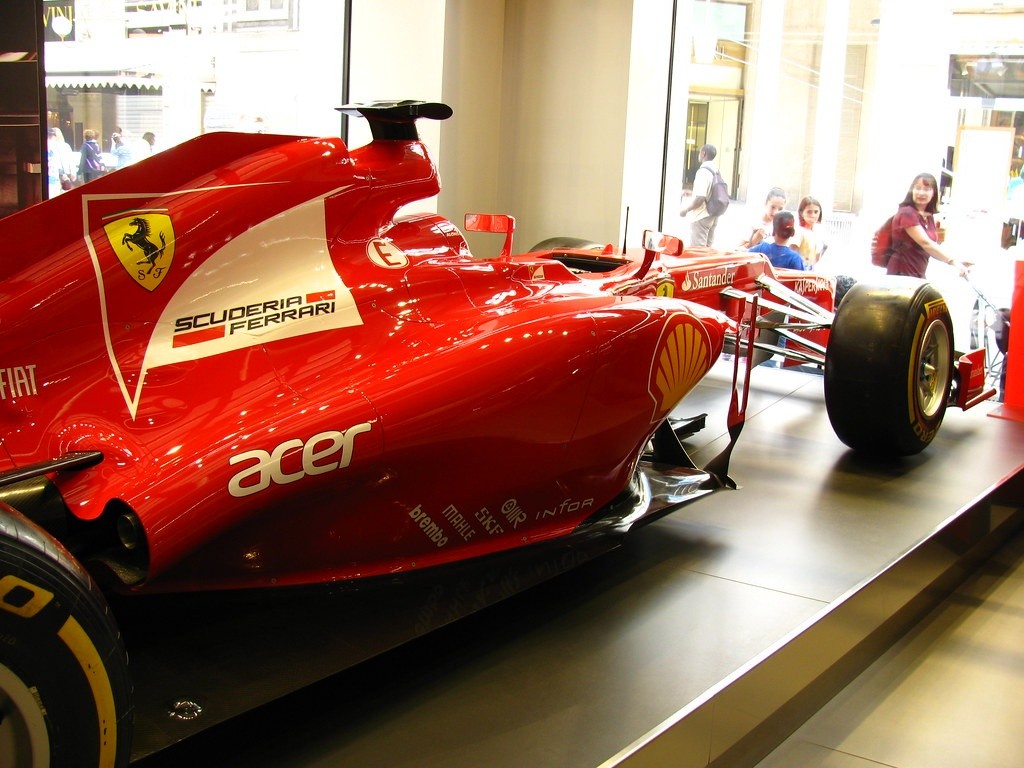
[962,269,1009,404]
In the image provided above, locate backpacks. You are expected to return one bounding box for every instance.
[86,142,107,171]
[872,215,894,267]
[700,165,729,216]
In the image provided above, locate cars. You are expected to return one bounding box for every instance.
[0,97,955,768]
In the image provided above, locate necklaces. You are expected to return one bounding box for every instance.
[918,211,929,229]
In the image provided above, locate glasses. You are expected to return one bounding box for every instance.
[66,123,71,125]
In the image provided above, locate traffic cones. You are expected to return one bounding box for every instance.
[985,261,1024,422]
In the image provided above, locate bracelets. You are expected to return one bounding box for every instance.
[684,208,688,214]
[948,258,954,265]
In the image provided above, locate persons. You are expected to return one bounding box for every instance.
[680,144,719,246]
[47,126,156,199]
[738,187,786,248]
[790,196,829,272]
[747,210,806,270]
[886,173,975,280]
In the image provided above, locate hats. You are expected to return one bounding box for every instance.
[112,133,121,138]
